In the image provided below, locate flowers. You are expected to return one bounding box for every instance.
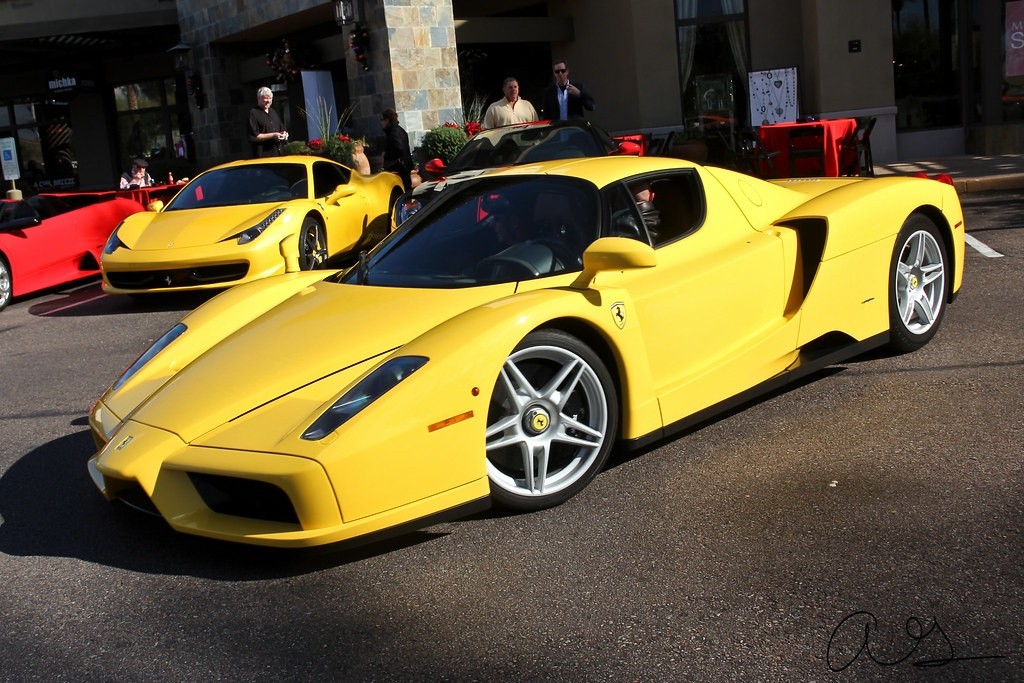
[466,122,483,136]
[310,137,324,151]
[443,121,461,130]
[338,135,351,145]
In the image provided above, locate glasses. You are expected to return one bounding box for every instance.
[494,216,502,222]
[380,118,384,121]
[554,69,566,73]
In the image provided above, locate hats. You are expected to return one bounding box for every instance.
[133,159,149,167]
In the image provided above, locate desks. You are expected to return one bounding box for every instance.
[759,118,856,178]
[39,182,189,200]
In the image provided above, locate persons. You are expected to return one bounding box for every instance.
[247,86,289,157]
[484,78,539,130]
[380,108,415,192]
[609,178,682,246]
[25,160,46,184]
[543,60,596,120]
[120,159,152,189]
[493,203,537,255]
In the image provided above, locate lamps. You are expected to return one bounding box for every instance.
[167,43,197,75]
[335,1,357,26]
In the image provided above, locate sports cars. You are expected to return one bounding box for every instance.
[85,165,965,550]
[393,121,656,234]
[99,155,405,296]
[0,189,152,309]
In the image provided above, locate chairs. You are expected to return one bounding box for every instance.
[786,124,825,179]
[716,122,777,180]
[840,117,878,177]
